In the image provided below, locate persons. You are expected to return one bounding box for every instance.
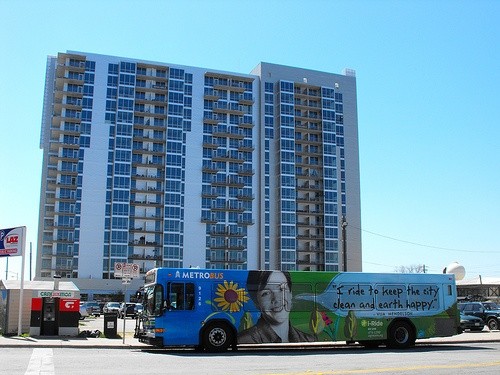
[237,270,318,345]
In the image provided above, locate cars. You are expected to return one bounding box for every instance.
[460,311,485,333]
[79,302,144,320]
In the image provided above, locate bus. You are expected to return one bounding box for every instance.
[133,266,461,354]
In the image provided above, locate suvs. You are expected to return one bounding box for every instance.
[457,301,500,330]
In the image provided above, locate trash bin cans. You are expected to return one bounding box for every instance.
[104,312,117,339]
